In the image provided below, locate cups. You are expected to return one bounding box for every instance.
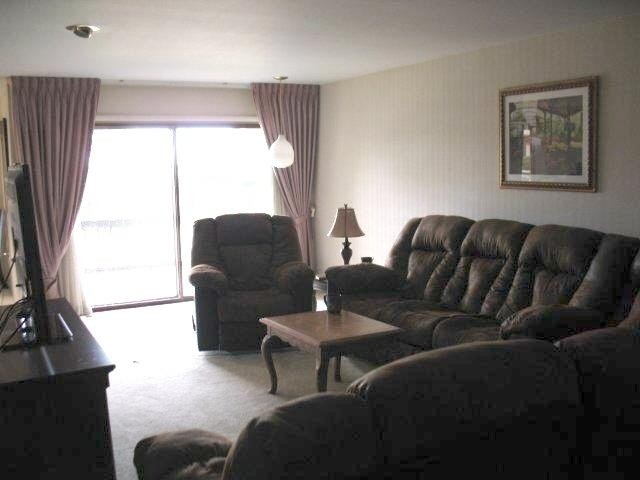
[361,256,375,263]
[323,294,343,314]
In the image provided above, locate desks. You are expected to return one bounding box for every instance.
[259,311,405,394]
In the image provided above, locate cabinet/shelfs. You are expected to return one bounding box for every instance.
[0,296,116,479]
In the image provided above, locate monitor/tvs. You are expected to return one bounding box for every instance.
[0,162,73,351]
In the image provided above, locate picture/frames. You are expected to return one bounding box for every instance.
[497,74,601,193]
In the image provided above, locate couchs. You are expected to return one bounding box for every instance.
[133,327,640,479]
[327,213,639,365]
[190,214,314,354]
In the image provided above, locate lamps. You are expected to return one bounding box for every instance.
[326,202,366,264]
